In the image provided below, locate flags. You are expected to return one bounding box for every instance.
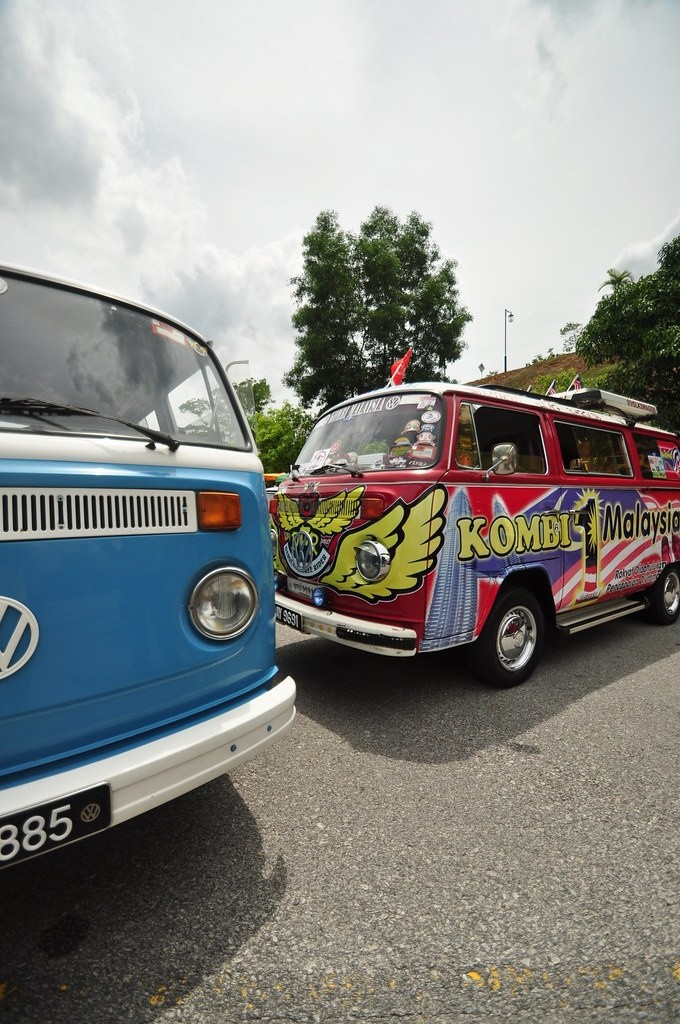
[548,380,556,395]
[573,375,582,390]
[391,348,412,386]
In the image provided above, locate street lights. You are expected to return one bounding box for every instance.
[504,308,514,374]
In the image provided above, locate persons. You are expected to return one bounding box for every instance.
[570,438,613,473]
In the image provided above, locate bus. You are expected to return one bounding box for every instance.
[268,380,680,689]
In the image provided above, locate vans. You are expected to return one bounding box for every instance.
[0,263,299,891]
